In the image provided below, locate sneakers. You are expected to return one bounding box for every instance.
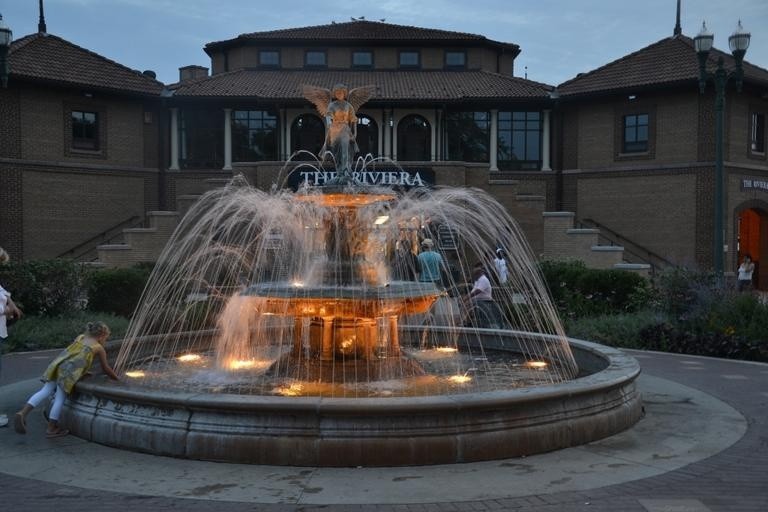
[45,423,70,438]
[0,414,9,427]
[14,411,27,434]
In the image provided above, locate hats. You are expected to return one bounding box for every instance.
[421,238,434,247]
[497,248,503,254]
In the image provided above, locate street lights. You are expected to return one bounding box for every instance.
[690,16,753,295]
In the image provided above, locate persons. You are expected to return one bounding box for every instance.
[455,267,492,328]
[492,248,510,288]
[303,83,376,187]
[0,247,27,426]
[737,253,755,292]
[418,238,447,292]
[391,237,419,282]
[14,320,123,438]
